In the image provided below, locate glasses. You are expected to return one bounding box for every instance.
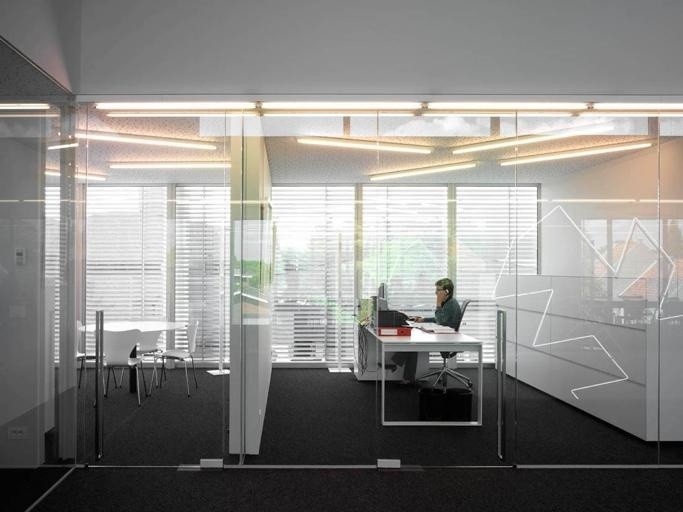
[437,288,444,292]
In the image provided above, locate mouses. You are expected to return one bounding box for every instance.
[409,318,414,320]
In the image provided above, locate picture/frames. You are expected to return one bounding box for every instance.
[261,198,273,288]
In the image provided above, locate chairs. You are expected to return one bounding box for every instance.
[414,298,471,394]
[119,329,167,389]
[75,320,118,398]
[92,329,147,408]
[149,320,200,398]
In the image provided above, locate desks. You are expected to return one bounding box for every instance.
[353,317,484,428]
[79,322,189,394]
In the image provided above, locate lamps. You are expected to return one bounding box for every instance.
[46,138,79,152]
[75,105,218,153]
[0,97,681,119]
[450,120,615,154]
[498,136,655,168]
[105,160,233,170]
[295,134,436,157]
[367,159,479,182]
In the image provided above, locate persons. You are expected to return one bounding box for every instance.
[379,279,461,386]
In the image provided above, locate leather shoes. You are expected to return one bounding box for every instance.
[378,362,397,372]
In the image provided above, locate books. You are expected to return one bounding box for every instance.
[415,323,457,334]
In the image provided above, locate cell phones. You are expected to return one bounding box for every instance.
[444,289,449,295]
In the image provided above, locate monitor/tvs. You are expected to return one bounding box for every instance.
[378,283,384,298]
